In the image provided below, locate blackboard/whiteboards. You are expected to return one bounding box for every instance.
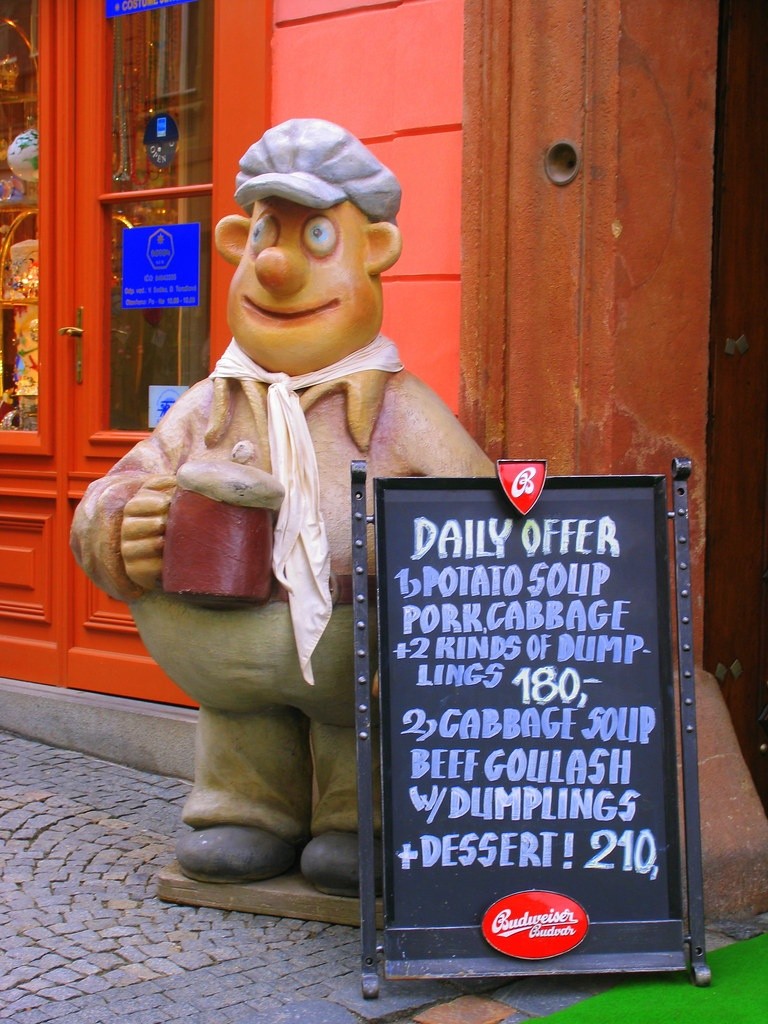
[371,472,685,931]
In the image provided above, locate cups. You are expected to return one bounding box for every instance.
[162,480,273,607]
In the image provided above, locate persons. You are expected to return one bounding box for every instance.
[68,118,497,898]
